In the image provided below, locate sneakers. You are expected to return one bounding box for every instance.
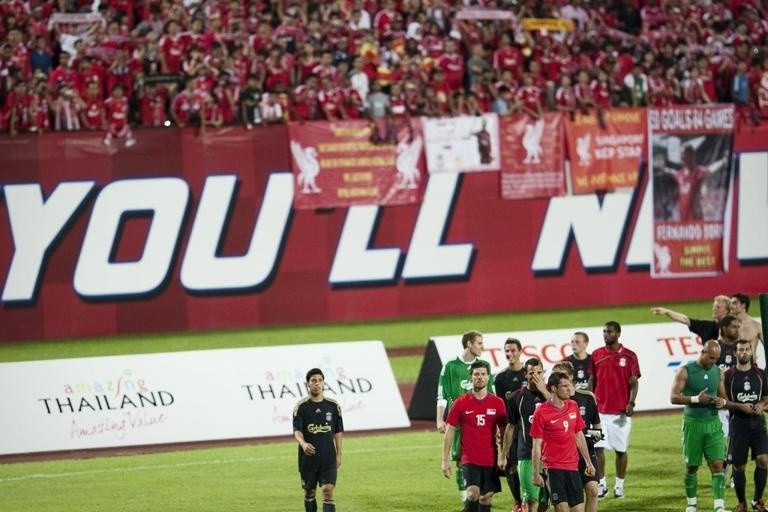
[614,485,625,500]
[512,502,522,512]
[597,485,608,500]
[751,499,768,512]
[733,505,747,512]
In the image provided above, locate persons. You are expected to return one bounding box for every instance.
[435,322,641,512]
[651,145,729,221]
[293,368,344,512]
[471,117,492,163]
[650,292,767,511]
